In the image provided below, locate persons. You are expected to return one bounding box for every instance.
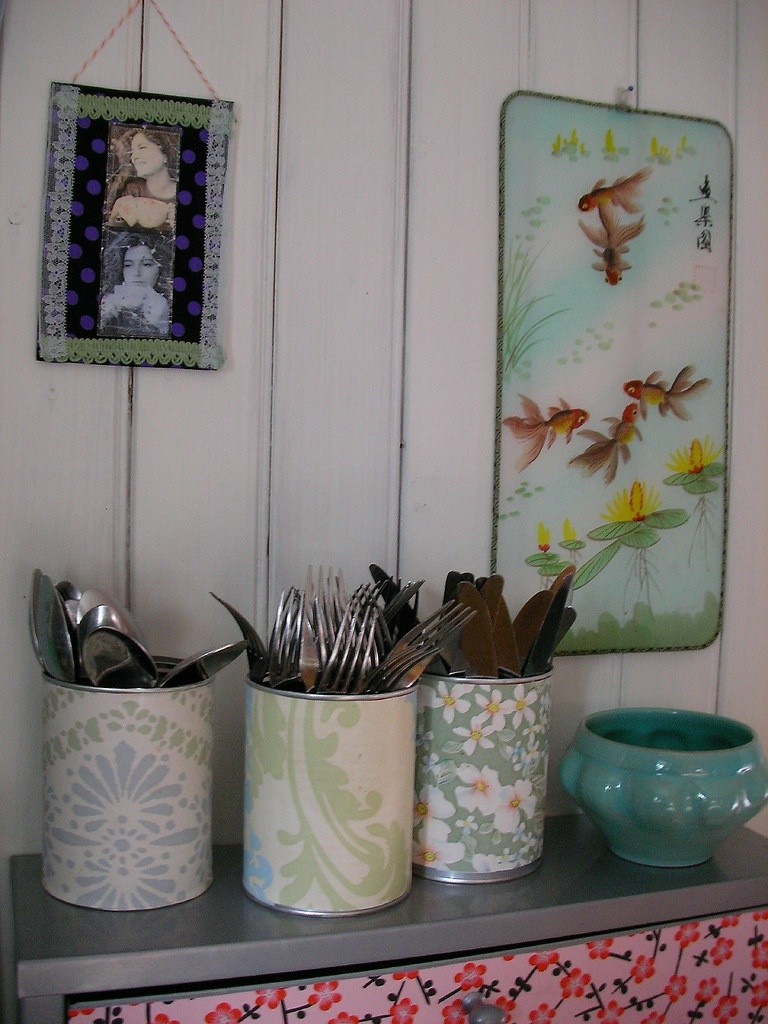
[109,124,178,228]
[98,233,170,336]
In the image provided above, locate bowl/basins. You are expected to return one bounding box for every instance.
[555,706,768,868]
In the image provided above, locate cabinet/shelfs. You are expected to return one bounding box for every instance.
[11,845,768,1023]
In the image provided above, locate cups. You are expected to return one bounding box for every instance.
[411,671,558,886]
[242,677,421,917]
[40,655,215,911]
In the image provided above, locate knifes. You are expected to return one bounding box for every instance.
[369,565,579,679]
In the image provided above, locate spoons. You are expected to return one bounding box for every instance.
[29,567,248,689]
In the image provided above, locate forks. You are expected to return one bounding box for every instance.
[207,565,478,696]
